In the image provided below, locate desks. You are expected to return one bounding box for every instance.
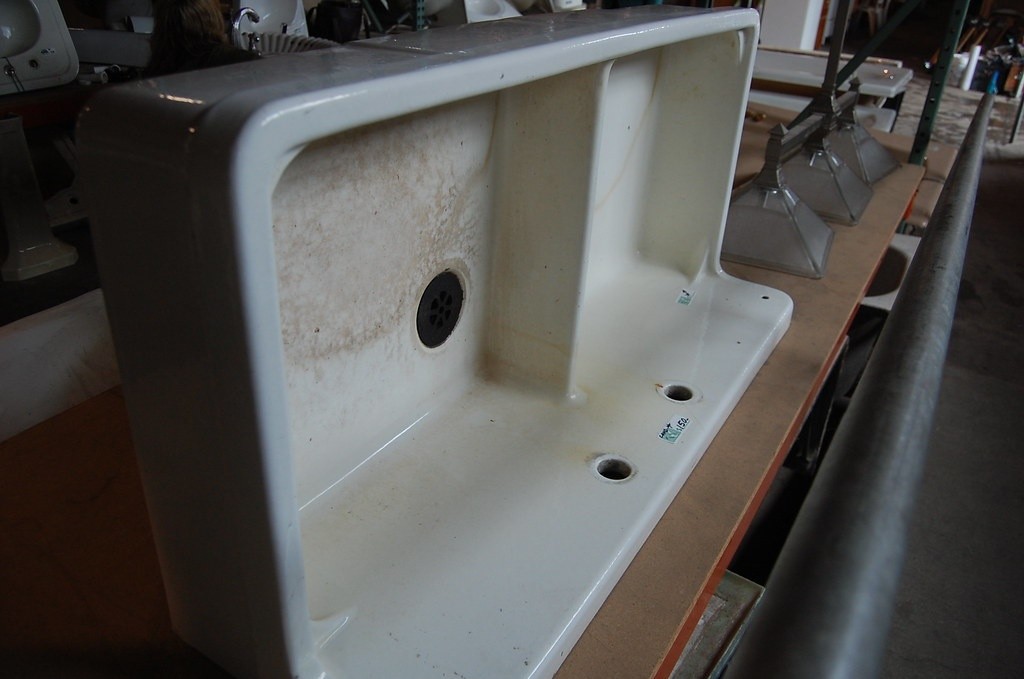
[750,50,914,106]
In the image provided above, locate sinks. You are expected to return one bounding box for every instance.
[242,0,308,37]
[602,5,795,496]
[466,0,523,24]
[72,49,610,667]
[550,0,590,10]
[0,0,77,97]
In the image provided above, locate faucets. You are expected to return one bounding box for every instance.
[229,8,260,54]
